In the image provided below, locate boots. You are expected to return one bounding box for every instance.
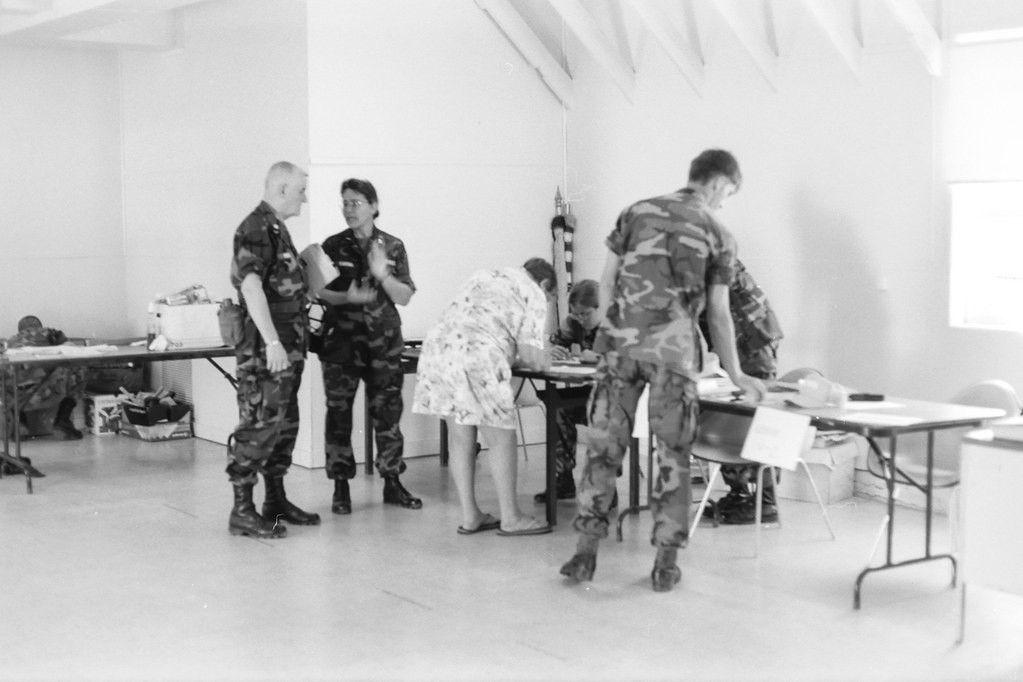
[229,483,287,538]
[652,552,681,591]
[534,472,576,502]
[609,488,618,510]
[262,477,321,526]
[560,554,595,580]
[332,480,351,515]
[53,398,83,440]
[703,487,778,523]
[383,474,422,509]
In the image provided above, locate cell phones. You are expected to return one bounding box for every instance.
[850,394,883,400]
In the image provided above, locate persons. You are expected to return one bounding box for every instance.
[560,149,765,593]
[698,259,784,525]
[534,280,624,512]
[315,179,422,513]
[412,257,553,535]
[225,161,320,539]
[6,316,89,440]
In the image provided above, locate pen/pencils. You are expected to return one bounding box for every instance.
[565,352,575,362]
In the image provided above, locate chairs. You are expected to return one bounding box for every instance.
[687,367,838,559]
[511,377,546,462]
[865,377,1023,569]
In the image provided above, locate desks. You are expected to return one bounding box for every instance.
[0,345,238,495]
[363,346,640,531]
[613,376,1008,610]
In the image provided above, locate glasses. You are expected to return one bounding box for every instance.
[343,199,366,207]
[567,310,591,322]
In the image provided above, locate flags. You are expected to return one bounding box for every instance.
[552,214,576,328]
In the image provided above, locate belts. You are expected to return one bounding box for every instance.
[269,300,302,314]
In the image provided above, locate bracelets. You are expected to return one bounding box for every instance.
[267,340,279,346]
[381,271,391,282]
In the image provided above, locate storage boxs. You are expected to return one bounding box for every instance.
[153,301,224,349]
[93,367,145,394]
[82,392,133,436]
[119,401,192,443]
[776,441,858,505]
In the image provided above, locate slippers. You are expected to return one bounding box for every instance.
[457,513,501,534]
[496,517,552,536]
